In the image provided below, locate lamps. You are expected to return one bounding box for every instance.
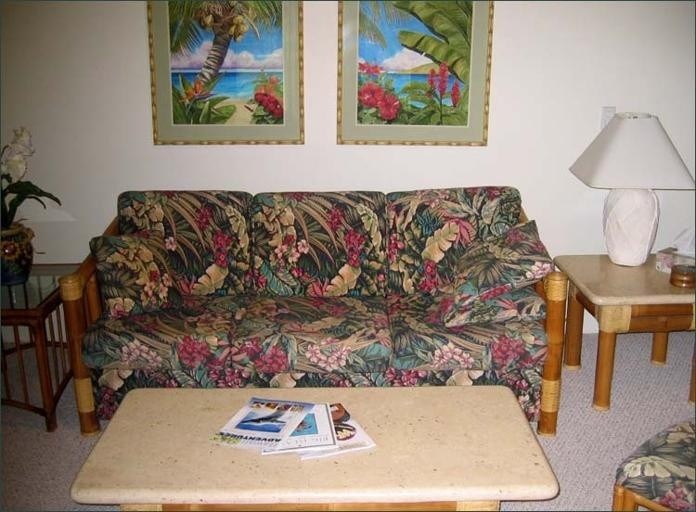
[571,108,695,267]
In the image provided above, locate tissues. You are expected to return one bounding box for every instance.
[655,228,695,272]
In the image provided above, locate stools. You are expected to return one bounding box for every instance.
[610,415,696,511]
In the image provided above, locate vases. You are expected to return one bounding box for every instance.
[1,224,34,286]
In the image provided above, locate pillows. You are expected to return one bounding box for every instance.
[434,220,555,327]
[87,231,187,320]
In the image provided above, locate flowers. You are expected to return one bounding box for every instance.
[253,90,284,125]
[358,61,468,126]
[0,126,61,228]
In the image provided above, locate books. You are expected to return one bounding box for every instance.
[209,396,377,461]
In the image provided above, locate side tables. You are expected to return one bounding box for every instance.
[554,252,696,411]
[0,260,82,433]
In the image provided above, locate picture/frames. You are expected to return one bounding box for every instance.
[336,2,495,149]
[145,1,305,147]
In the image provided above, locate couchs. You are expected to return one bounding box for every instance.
[60,185,569,438]
